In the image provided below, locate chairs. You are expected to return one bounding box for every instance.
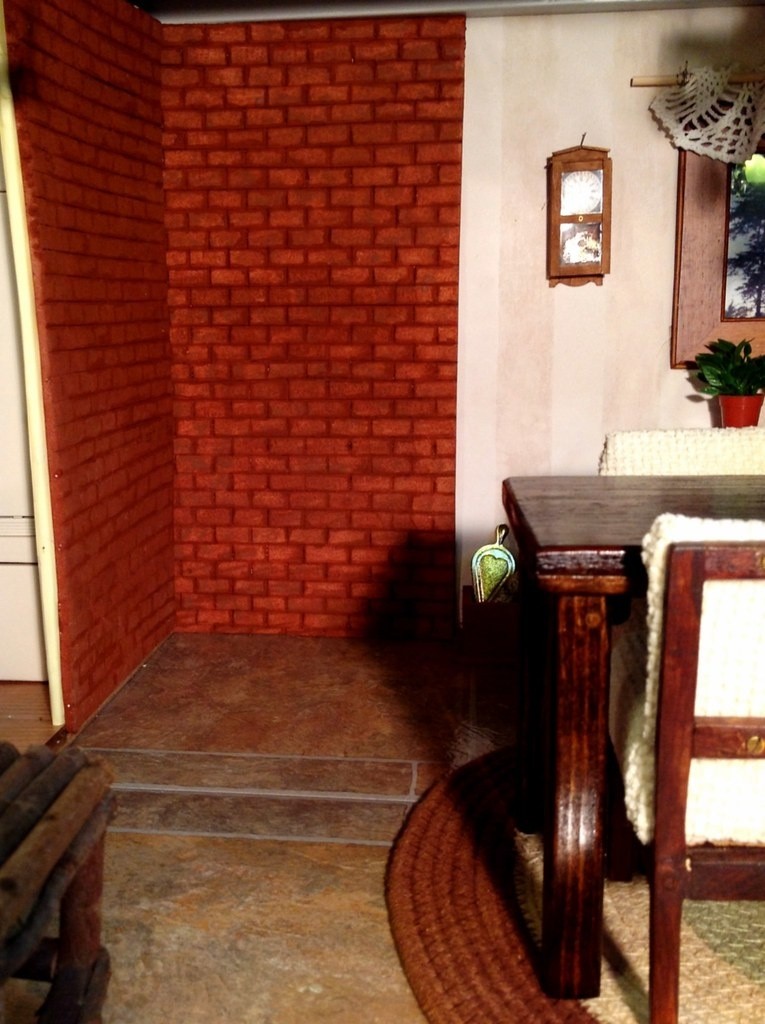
[610,540,764,1024]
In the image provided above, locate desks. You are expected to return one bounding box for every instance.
[502,475,765,1000]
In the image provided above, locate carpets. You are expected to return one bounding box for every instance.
[385,747,764,1024]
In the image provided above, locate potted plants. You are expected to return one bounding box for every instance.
[695,338,765,427]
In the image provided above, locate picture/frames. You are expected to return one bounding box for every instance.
[671,102,765,369]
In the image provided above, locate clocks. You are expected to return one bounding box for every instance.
[562,171,602,213]
